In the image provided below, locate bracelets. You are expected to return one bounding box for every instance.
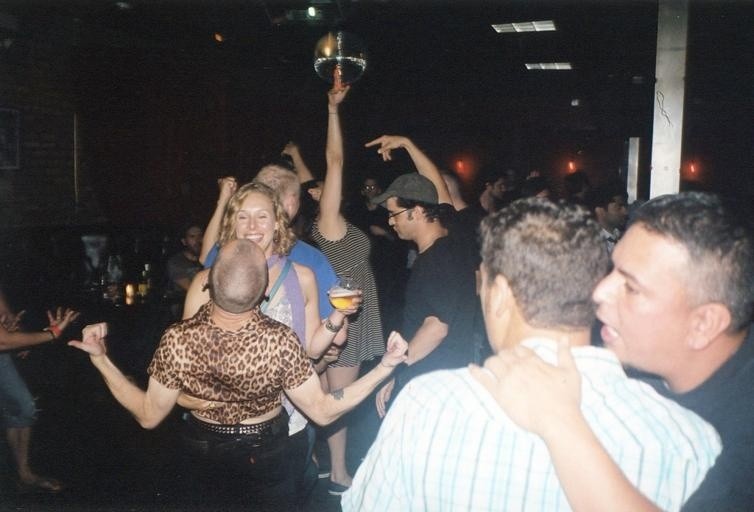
[327,112,338,115]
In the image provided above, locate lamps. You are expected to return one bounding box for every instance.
[313,22,368,86]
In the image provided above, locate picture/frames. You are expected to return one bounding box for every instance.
[0,107,21,170]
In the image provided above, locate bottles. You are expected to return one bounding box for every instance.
[124,262,154,301]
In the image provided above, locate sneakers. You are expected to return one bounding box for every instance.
[317,466,349,497]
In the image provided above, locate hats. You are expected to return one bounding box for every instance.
[371,170,438,206]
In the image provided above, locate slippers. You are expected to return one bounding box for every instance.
[15,473,63,496]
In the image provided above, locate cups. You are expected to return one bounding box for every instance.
[329,277,363,310]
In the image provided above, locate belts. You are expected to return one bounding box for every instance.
[190,406,286,437]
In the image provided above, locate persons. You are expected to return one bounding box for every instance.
[1,295,80,492]
[297,63,387,497]
[466,163,628,230]
[67,134,482,512]
[339,186,723,511]
[465,193,754,511]
